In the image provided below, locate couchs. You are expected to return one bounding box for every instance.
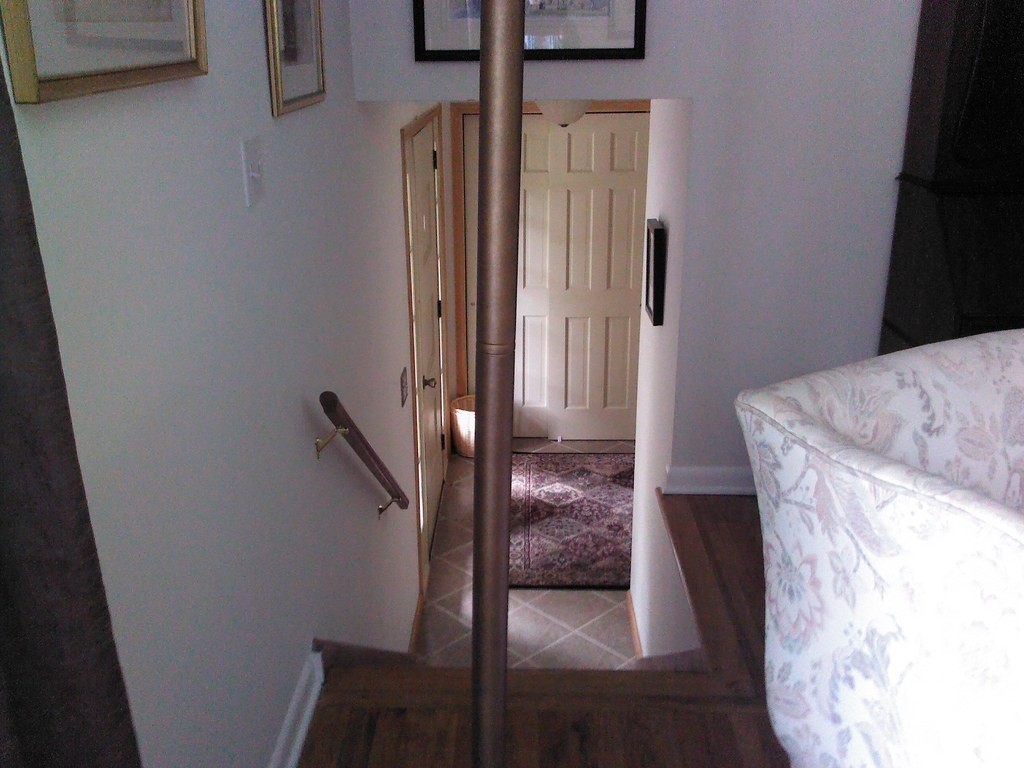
[734,328,1024,768]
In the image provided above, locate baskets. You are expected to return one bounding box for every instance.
[450,394,476,458]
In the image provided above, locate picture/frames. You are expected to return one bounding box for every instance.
[264,0,326,117]
[645,219,666,326]
[0,0,208,105]
[413,0,646,61]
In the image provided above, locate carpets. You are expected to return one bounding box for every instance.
[508,451,635,590]
